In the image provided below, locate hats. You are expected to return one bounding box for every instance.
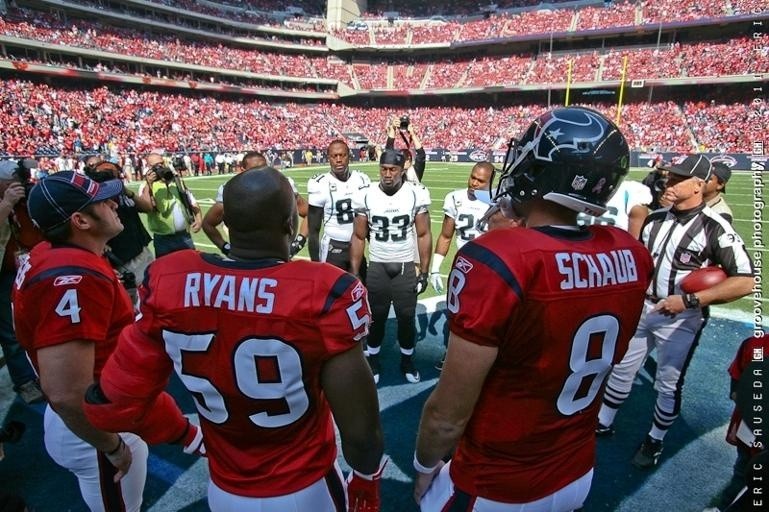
[0,159,20,178]
[710,161,732,184]
[27,169,123,231]
[656,153,712,179]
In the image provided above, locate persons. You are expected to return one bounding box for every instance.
[12,170,208,511]
[0,149,760,468]
[704,329,768,512]
[407,107,655,511]
[0,1,375,182]
[370,0,769,157]
[82,164,387,512]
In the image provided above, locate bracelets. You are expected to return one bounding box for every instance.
[107,437,126,461]
[411,450,446,477]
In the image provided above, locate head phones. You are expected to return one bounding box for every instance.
[92,160,124,183]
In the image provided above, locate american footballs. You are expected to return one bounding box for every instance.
[680,267,727,293]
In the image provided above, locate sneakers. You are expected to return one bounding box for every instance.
[631,434,665,470]
[368,359,381,383]
[594,422,617,437]
[399,360,421,384]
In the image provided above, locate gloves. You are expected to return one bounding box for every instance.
[343,471,381,512]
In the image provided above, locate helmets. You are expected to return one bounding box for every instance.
[501,105,631,218]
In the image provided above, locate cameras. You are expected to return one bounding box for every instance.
[102,249,124,269]
[120,271,136,290]
[399,115,410,133]
[149,162,174,181]
[16,157,36,201]
